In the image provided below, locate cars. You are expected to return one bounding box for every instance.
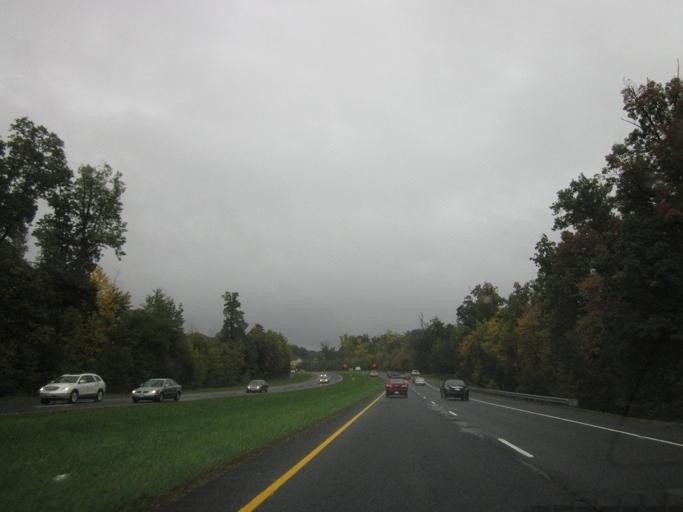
[37,373,106,405]
[439,378,470,401]
[129,376,184,404]
[385,375,410,397]
[246,379,269,394]
